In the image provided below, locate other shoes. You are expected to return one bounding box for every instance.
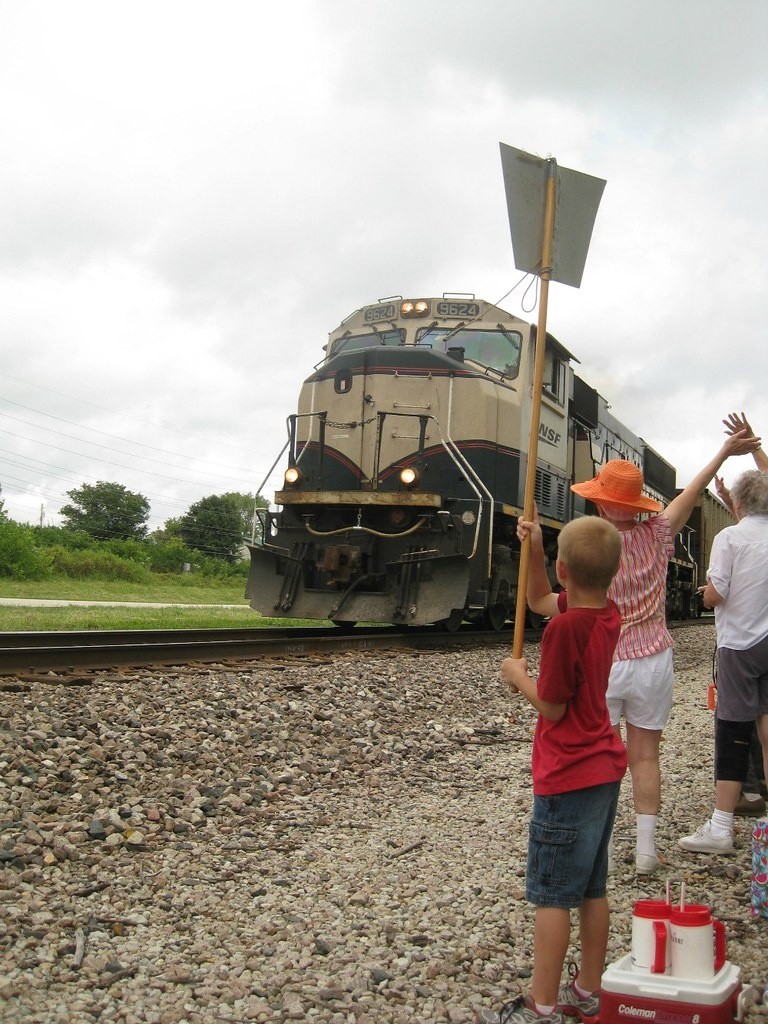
[734,797,765,818]
[635,853,658,875]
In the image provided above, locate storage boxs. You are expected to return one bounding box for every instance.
[752,815,768,918]
[597,953,755,1024]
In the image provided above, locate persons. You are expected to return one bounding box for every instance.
[678,413,767,855]
[473,514,628,1022]
[572,428,762,878]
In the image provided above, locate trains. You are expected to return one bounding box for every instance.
[244,291,737,633]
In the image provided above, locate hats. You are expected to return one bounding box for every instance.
[571,459,662,513]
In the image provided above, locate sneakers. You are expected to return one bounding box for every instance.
[472,993,564,1024]
[678,819,733,854]
[557,962,600,1024]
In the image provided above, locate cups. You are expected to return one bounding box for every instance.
[631,899,676,977]
[671,904,726,982]
